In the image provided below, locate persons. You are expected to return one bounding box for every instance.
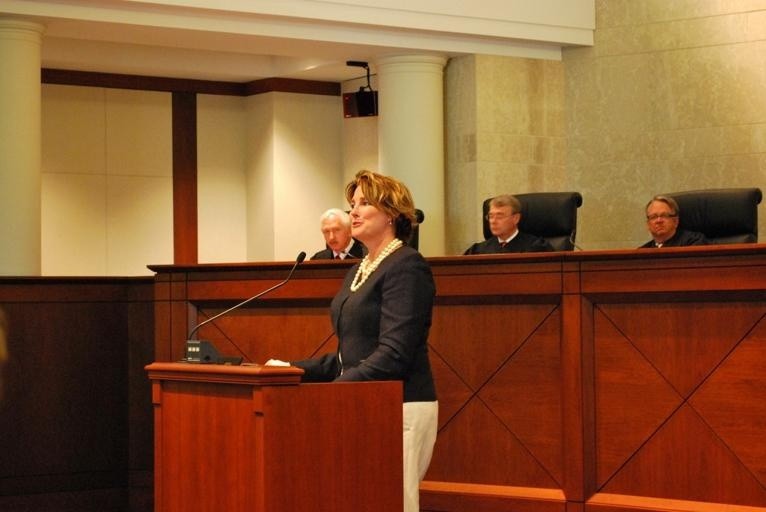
[462,195,554,255]
[309,207,366,260]
[267,169,439,511]
[639,193,713,248]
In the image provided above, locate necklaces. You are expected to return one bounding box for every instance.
[349,237,405,293]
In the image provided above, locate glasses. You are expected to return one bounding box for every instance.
[484,211,512,221]
[646,214,677,221]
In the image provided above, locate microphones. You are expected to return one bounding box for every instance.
[188,252,306,340]
[569,229,582,251]
[341,249,356,259]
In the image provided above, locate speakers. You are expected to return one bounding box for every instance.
[343,91,378,118]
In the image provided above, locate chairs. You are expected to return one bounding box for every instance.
[650,187,760,245]
[479,189,584,248]
